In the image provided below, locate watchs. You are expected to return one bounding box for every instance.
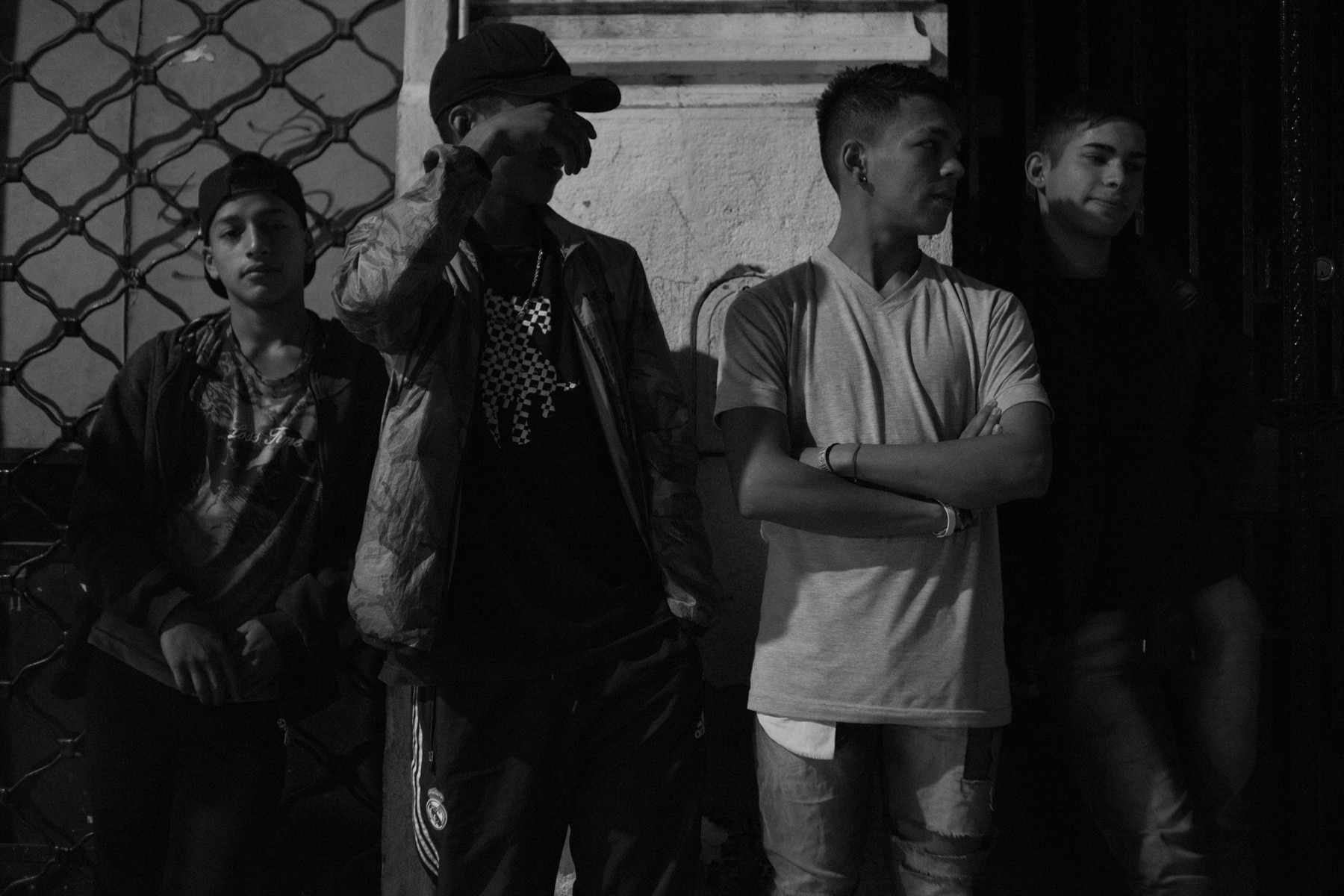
[954,508,974,532]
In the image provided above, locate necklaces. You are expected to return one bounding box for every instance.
[484,239,542,324]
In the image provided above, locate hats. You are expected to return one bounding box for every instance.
[428,23,622,121]
[197,152,317,300]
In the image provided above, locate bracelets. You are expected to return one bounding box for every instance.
[818,442,840,473]
[852,442,862,482]
[925,496,956,538]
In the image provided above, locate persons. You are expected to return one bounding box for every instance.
[66,152,388,896]
[964,93,1262,896]
[330,22,717,896]
[711,60,1054,896]
